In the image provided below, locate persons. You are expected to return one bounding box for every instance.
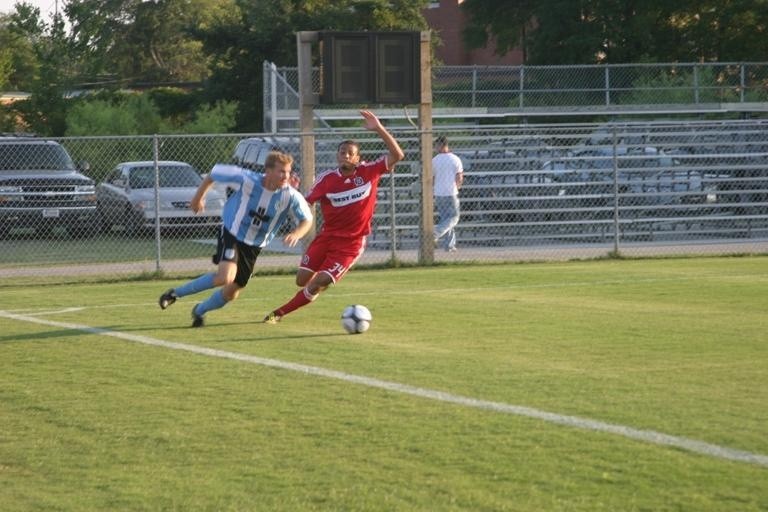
[430,136,464,253]
[261,110,406,325]
[159,150,313,325]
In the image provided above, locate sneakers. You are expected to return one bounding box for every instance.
[159,288,177,309]
[264,312,281,324]
[432,231,439,248]
[445,247,457,252]
[191,304,204,325]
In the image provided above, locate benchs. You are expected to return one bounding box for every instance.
[279,118,764,250]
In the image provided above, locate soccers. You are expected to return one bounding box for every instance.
[341,304,371,333]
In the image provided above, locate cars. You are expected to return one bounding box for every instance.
[232,136,335,174]
[0,137,226,240]
